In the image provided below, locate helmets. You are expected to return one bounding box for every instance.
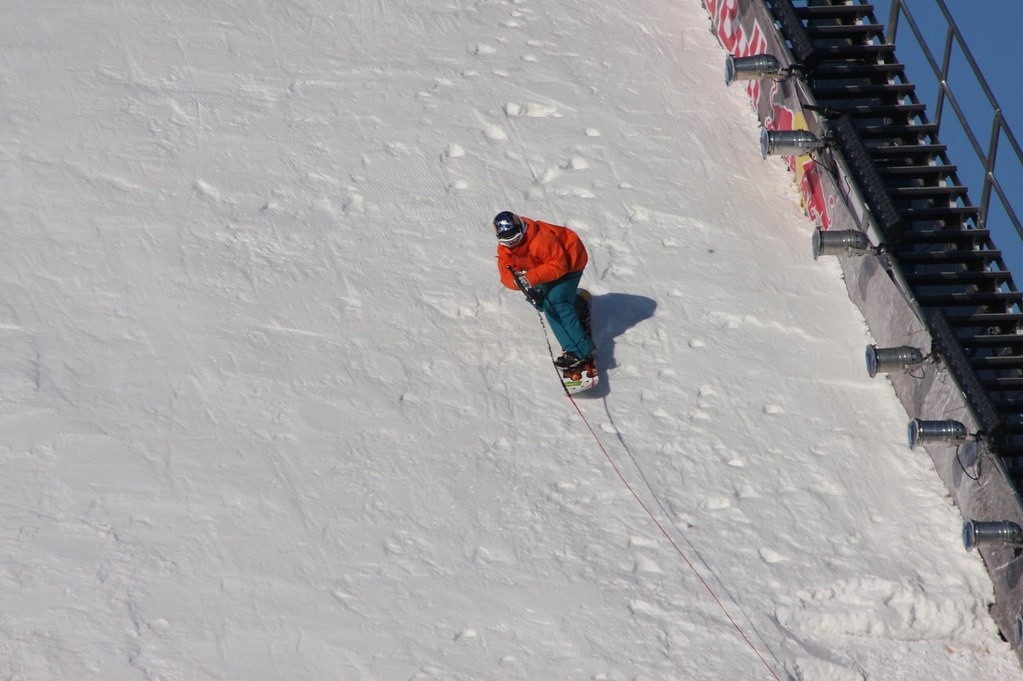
[493,211,525,239]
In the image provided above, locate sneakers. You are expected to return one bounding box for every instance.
[554,353,587,370]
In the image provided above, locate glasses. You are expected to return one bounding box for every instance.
[498,233,523,248]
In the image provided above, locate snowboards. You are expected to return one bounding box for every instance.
[563,288,598,395]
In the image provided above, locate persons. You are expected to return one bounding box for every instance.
[493,211,593,370]
